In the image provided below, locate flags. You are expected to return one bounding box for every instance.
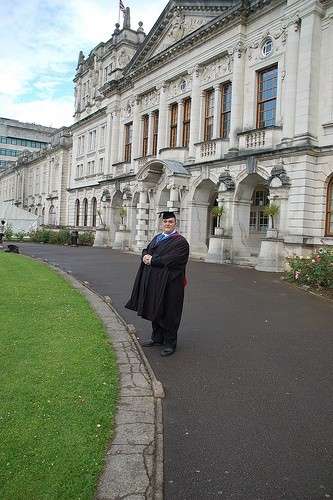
[119,0,126,13]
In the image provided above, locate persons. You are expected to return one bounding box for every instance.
[124,211,189,357]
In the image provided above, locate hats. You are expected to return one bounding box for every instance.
[157,211,180,220]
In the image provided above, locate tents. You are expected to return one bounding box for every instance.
[0,203,39,234]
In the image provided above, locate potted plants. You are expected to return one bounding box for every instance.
[260,203,281,239]
[118,207,127,230]
[212,205,226,235]
[95,209,106,230]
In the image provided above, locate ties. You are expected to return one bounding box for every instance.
[155,234,165,245]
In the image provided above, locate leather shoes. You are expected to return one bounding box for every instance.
[141,340,162,346]
[162,346,175,357]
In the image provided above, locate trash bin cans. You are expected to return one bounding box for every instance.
[71,231,79,247]
